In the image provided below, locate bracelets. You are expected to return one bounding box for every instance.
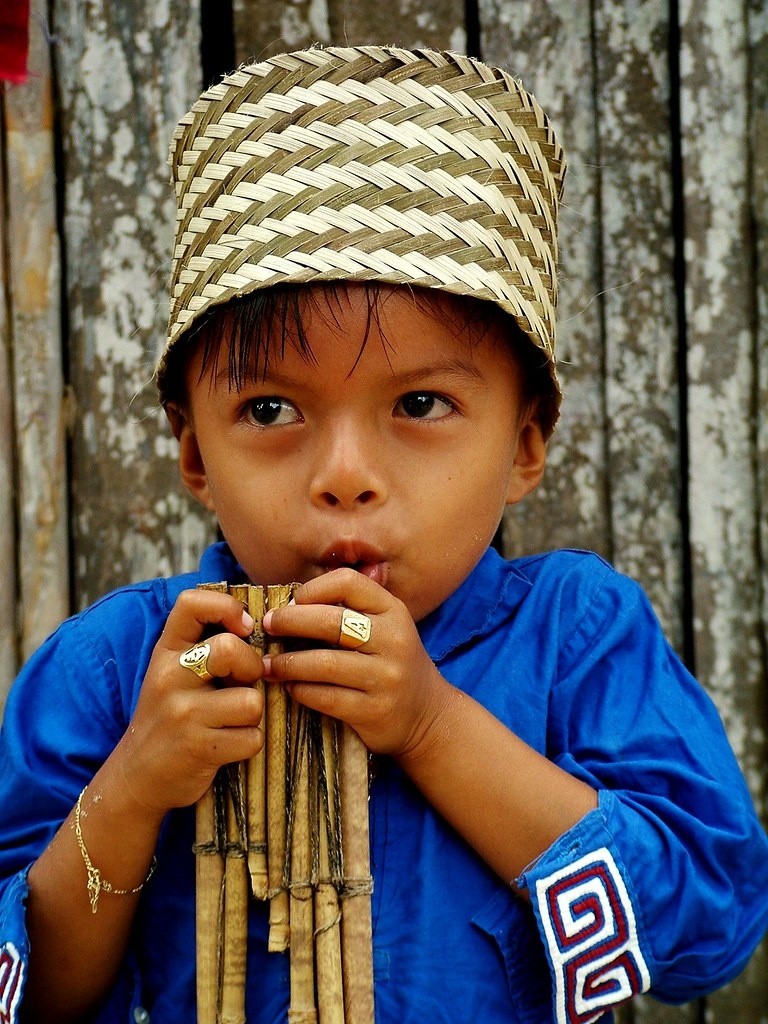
[75,786,157,913]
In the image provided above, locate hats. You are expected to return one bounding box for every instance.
[153,46,565,426]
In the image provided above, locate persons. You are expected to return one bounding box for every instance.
[0,45,768,1024]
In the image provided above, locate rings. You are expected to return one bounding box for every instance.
[338,608,372,647]
[179,641,214,682]
